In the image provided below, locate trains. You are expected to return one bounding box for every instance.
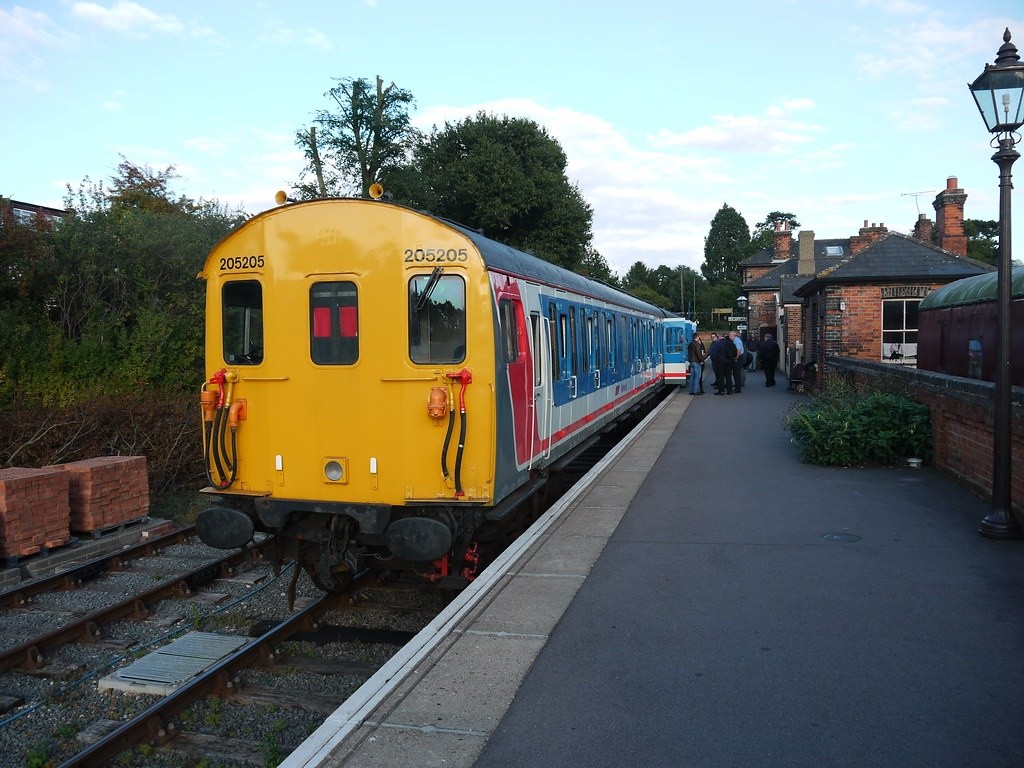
[194,183,699,617]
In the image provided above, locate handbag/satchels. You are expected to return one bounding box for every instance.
[734,351,754,368]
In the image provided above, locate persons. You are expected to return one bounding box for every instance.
[678,334,689,351]
[760,334,780,387]
[746,333,759,372]
[710,331,746,395]
[687,333,706,396]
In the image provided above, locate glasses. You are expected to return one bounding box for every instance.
[711,337,715,338]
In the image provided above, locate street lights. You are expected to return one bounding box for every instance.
[968,24,1024,543]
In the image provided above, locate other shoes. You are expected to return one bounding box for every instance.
[726,391,732,396]
[733,389,742,394]
[711,383,717,386]
[701,391,706,394]
[714,391,725,395]
[765,382,776,387]
[689,393,694,395]
[714,386,718,390]
[695,393,703,395]
